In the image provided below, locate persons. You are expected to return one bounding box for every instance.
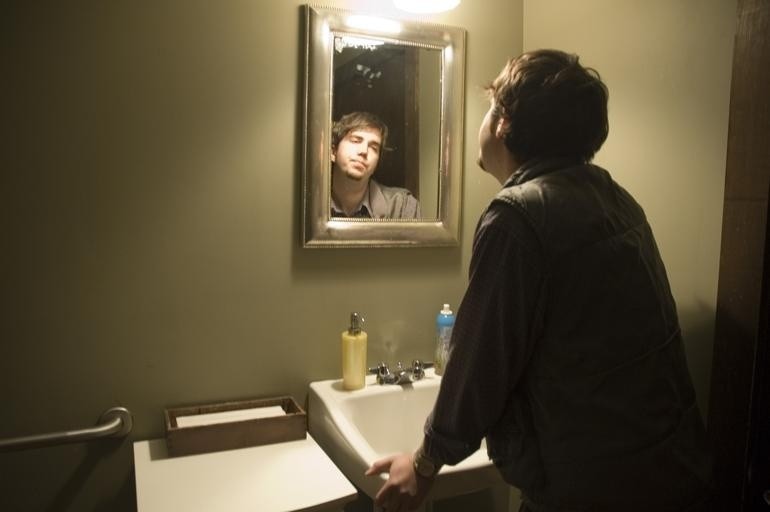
[327,107,424,223]
[362,48,734,511]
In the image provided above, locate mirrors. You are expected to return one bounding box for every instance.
[303,3,468,247]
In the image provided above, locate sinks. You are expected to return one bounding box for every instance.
[334,377,491,468]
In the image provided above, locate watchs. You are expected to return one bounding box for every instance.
[412,451,438,483]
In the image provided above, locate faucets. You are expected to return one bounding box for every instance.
[392,360,415,384]
